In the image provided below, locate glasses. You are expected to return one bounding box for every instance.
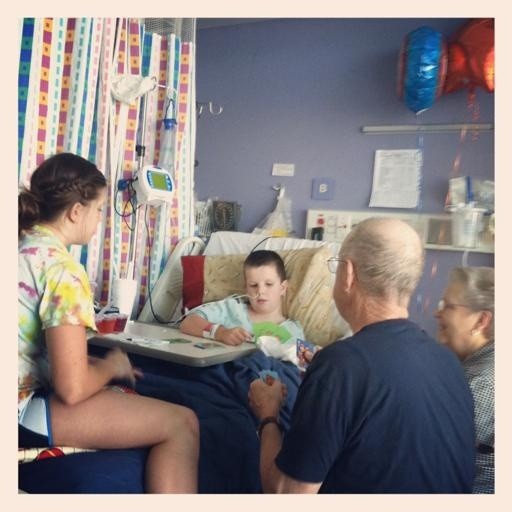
[327,257,358,283]
[437,298,469,313]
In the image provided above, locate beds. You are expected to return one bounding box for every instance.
[18,231,356,494]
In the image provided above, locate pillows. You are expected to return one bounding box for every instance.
[182,247,336,346]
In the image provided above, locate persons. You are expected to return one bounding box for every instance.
[179,249,304,379]
[430,262,495,492]
[19,151,204,493]
[247,217,479,493]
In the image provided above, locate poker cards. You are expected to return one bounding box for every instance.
[296,339,314,369]
[258,368,279,387]
[124,338,190,347]
[252,322,293,344]
[194,342,224,350]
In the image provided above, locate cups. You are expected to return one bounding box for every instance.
[450,207,487,248]
[93,313,128,334]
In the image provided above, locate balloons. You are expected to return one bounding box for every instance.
[443,20,494,97]
[393,26,449,116]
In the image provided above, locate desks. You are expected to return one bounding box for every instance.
[87,320,257,367]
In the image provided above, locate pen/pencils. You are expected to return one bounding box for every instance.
[112,259,134,281]
[126,338,172,345]
[467,175,472,205]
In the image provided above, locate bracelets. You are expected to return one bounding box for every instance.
[201,321,223,339]
[253,415,279,441]
